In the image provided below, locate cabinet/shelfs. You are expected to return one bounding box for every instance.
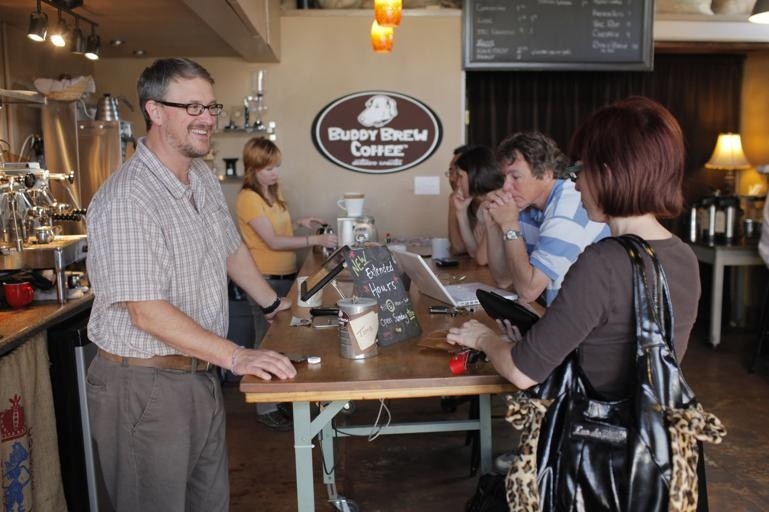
[208,128,274,184]
[0,0,282,63]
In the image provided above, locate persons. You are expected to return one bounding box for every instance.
[452,146,503,266]
[480,128,613,310]
[85,57,298,512]
[445,143,484,256]
[236,136,338,430]
[446,94,727,511]
[757,192,769,271]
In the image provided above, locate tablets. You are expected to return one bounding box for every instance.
[301,244,352,301]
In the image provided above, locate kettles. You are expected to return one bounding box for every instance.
[309,223,336,257]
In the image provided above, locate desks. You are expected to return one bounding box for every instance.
[675,234,765,353]
[238,244,519,512]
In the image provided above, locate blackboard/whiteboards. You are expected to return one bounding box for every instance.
[461,0,655,72]
[343,245,423,347]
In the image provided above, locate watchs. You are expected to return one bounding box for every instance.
[503,231,523,241]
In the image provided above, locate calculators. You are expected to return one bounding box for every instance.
[312,315,340,328]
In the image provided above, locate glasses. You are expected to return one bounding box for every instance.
[146,96,225,118]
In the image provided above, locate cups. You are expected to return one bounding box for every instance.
[337,191,364,218]
[34,224,56,245]
[67,274,84,288]
[222,157,238,177]
[431,238,451,260]
[2,279,34,310]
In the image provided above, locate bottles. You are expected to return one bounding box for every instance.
[384,233,391,245]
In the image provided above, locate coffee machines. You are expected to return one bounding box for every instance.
[335,215,380,269]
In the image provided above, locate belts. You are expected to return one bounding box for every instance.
[95,346,217,374]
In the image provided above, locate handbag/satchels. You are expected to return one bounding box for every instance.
[529,229,729,512]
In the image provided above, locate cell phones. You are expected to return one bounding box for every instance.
[436,258,460,267]
[279,352,309,363]
[309,307,339,316]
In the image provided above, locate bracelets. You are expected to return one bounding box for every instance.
[231,346,245,377]
[306,236,309,248]
[263,298,281,314]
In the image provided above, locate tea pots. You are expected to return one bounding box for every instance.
[94,92,134,122]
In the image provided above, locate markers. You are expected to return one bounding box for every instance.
[453,306,474,312]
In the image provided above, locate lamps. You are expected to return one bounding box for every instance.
[703,132,751,195]
[747,0,769,24]
[370,20,395,55]
[372,0,404,28]
[26,0,103,65]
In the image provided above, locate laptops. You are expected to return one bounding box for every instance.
[393,249,519,306]
